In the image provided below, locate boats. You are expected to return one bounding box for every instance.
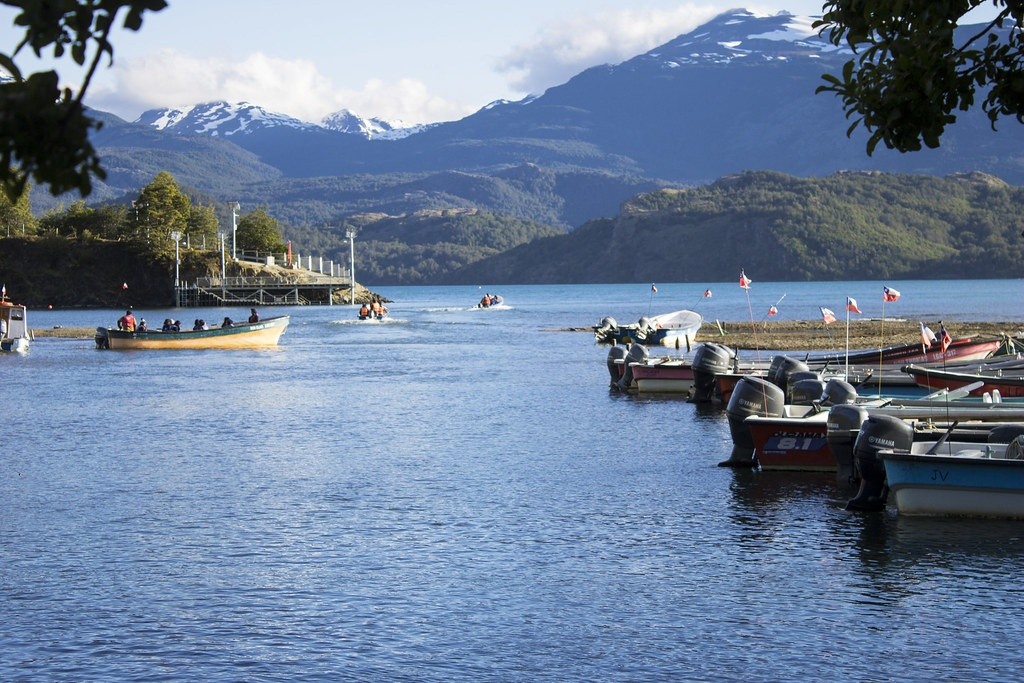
[357,304,390,322]
[93,313,291,351]
[590,310,703,348]
[607,325,1024,525]
[477,294,505,309]
[0,284,32,353]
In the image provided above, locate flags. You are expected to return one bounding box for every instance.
[740,272,752,289]
[768,306,778,316]
[704,289,712,298]
[882,286,900,303]
[921,323,938,349]
[820,307,837,325]
[940,324,952,352]
[652,285,657,292]
[847,297,862,314]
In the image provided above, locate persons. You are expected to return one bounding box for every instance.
[360,295,387,319]
[162,319,182,333]
[137,318,147,332]
[481,292,500,307]
[249,307,260,324]
[222,316,235,328]
[193,318,208,330]
[117,310,136,331]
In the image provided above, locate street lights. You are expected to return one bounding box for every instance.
[344,229,358,305]
[227,201,241,260]
[217,230,228,300]
[170,230,182,287]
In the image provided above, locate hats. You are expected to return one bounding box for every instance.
[140,318,145,322]
